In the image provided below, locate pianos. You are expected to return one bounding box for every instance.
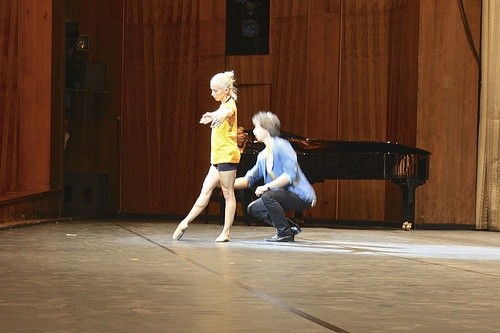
[237,130,432,229]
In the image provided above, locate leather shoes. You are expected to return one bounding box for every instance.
[291,224,302,235]
[264,233,294,242]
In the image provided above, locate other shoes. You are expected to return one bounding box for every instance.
[173,221,188,240]
[216,237,229,242]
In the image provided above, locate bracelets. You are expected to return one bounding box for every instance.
[266,183,270,190]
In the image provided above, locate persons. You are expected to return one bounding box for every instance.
[173,70,240,242]
[237,127,247,153]
[234,110,317,243]
